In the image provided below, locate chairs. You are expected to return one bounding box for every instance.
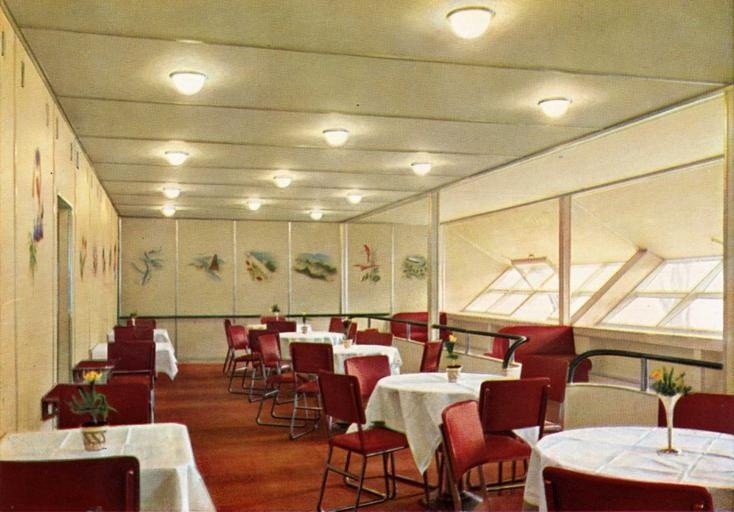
[0,316,156,512]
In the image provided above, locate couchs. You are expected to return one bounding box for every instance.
[391,311,453,342]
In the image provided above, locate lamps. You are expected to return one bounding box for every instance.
[445,6,496,40]
[538,97,573,118]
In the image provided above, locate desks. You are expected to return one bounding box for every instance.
[92,342,178,380]
[0,423,217,512]
[106,327,175,351]
